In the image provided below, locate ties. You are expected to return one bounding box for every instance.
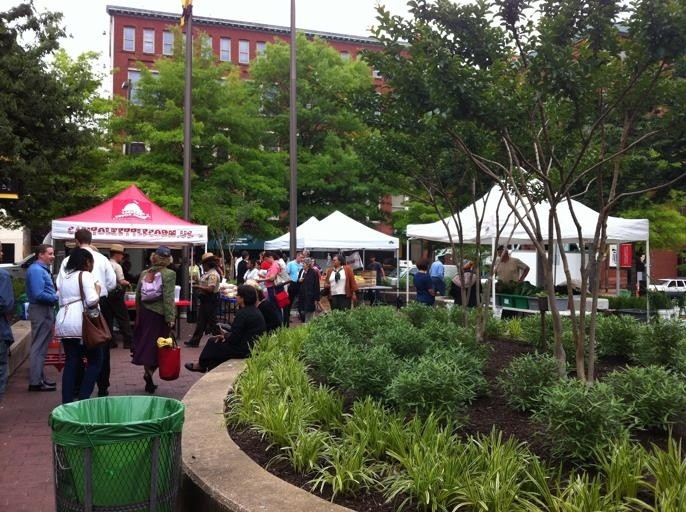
[46,267,58,293]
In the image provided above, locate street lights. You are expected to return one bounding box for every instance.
[121,79,134,155]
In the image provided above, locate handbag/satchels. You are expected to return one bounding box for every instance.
[140,271,163,302]
[274,288,291,309]
[82,311,112,351]
[158,328,180,381]
[273,267,292,288]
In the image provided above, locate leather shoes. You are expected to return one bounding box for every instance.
[42,379,55,386]
[29,383,56,391]
[184,362,207,374]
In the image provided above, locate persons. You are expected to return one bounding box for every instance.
[184,285,268,373]
[495,245,531,320]
[430,256,446,303]
[0,229,184,403]
[366,251,387,307]
[181,244,359,348]
[449,270,483,308]
[457,252,482,273]
[636,252,650,296]
[412,256,437,306]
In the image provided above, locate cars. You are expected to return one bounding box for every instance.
[649,279,686,293]
[385,265,417,286]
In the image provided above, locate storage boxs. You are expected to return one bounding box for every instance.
[361,270,377,286]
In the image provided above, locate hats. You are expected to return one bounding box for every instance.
[110,243,124,252]
[156,246,170,257]
[201,252,215,264]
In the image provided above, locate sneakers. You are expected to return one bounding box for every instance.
[144,373,158,394]
[184,340,200,347]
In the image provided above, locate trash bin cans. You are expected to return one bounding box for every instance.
[597,308,659,324]
[48,395,186,512]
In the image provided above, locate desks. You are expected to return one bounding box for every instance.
[358,286,393,306]
[124,300,190,339]
[219,297,237,325]
